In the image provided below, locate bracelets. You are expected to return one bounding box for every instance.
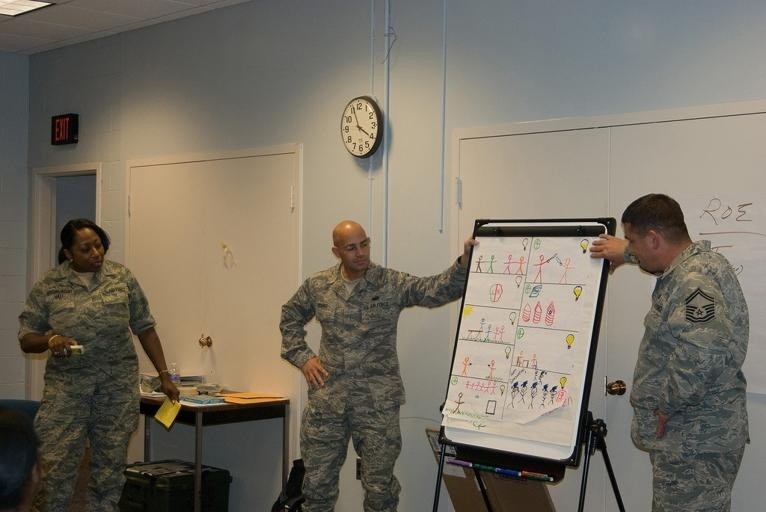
[48,334,61,343]
[159,369,168,376]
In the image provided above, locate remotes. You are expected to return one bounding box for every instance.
[61,342,85,360]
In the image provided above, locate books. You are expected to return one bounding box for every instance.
[153,397,181,429]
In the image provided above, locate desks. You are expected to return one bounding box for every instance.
[136,385,288,512]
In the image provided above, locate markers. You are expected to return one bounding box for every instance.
[446,459,554,482]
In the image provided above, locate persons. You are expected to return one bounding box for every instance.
[16,217,184,511]
[587,193,751,512]
[279,221,480,511]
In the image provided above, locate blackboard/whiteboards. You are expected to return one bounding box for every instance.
[438,217,617,467]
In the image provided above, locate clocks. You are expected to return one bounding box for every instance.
[339,97,382,160]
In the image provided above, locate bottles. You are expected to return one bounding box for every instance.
[168,362,181,389]
